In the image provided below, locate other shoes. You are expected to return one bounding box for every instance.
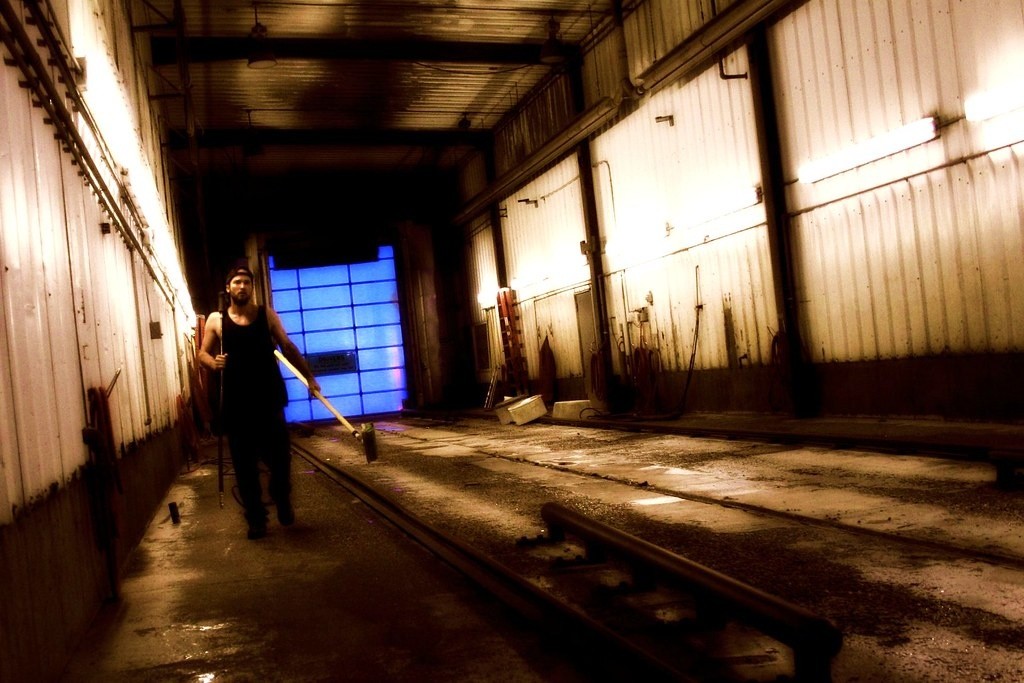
[247,525,265,540]
[277,501,293,526]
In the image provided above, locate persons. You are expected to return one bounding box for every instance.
[198,265,320,539]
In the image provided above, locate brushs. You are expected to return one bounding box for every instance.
[273,349,379,464]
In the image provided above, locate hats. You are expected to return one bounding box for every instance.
[226,264,254,285]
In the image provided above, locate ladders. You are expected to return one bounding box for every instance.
[496,288,530,395]
